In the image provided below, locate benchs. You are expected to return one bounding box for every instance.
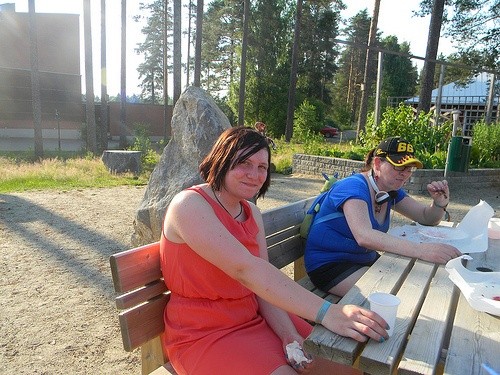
[110,193,342,375]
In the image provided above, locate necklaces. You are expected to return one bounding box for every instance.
[212,185,242,219]
[375,205,381,213]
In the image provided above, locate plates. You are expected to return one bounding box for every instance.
[418,227,470,241]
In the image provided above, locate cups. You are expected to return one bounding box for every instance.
[368,292,401,337]
[487,217,500,239]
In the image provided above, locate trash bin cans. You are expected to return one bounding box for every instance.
[444,137,472,176]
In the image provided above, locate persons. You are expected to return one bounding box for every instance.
[304,137,462,297]
[159,125,391,375]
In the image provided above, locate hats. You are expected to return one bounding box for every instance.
[373,137,423,169]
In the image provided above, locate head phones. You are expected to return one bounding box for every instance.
[367,169,396,205]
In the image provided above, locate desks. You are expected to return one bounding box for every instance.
[303,220,500,375]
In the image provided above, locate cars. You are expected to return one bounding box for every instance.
[320,125,338,138]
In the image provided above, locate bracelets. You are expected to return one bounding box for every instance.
[433,202,448,209]
[315,300,332,325]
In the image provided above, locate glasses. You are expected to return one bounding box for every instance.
[384,159,417,173]
